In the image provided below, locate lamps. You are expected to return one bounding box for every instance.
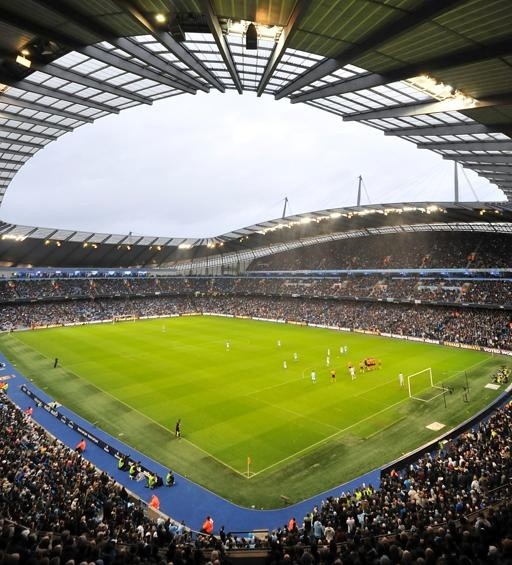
[169,17,187,44]
[247,18,259,50]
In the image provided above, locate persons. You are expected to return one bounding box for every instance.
[331,368,336,383]
[1,237,512,355]
[53,358,58,368]
[226,535,270,550]
[491,364,512,384]
[270,398,512,565]
[358,357,383,375]
[326,356,330,367]
[399,372,405,386]
[294,353,298,360]
[283,360,288,368]
[349,366,357,380]
[0,391,226,565]
[311,370,317,384]
